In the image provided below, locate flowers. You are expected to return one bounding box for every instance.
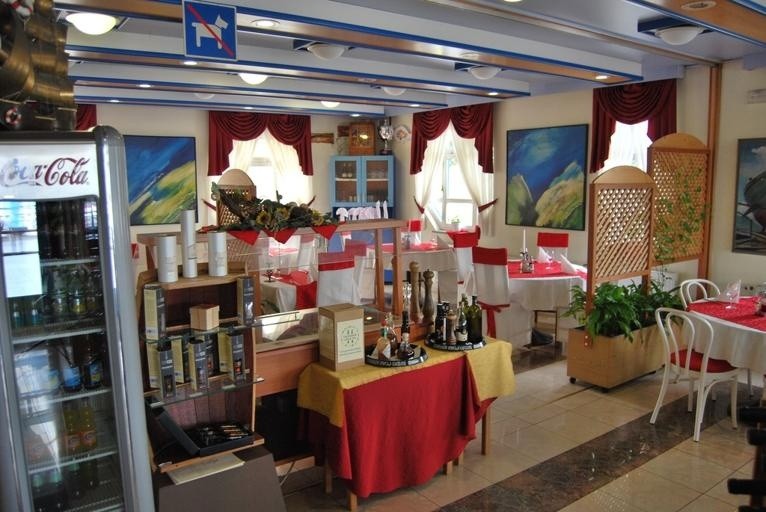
[208,182,340,234]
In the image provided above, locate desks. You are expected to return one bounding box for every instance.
[297,332,516,512]
[157,449,286,512]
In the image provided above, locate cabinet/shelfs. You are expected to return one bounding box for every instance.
[135,217,409,477]
[328,154,395,220]
[134,259,266,474]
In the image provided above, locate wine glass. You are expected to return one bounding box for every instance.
[726,282,738,309]
[544,248,555,269]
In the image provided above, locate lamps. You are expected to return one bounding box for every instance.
[293,40,356,62]
[56,10,131,36]
[321,101,340,108]
[237,72,268,85]
[194,92,215,100]
[637,16,715,46]
[453,62,507,80]
[370,84,406,96]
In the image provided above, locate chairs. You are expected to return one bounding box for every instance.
[679,278,721,311]
[650,307,742,441]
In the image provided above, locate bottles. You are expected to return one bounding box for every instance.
[342,163,385,203]
[1,203,108,510]
[520,255,535,273]
[436,291,484,345]
[376,309,414,360]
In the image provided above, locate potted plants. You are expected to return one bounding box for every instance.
[559,277,692,394]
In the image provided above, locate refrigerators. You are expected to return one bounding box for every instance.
[0,125,156,509]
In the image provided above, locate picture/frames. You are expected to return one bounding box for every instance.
[731,137,766,256]
[394,126,410,142]
[504,123,588,231]
[121,134,197,226]
